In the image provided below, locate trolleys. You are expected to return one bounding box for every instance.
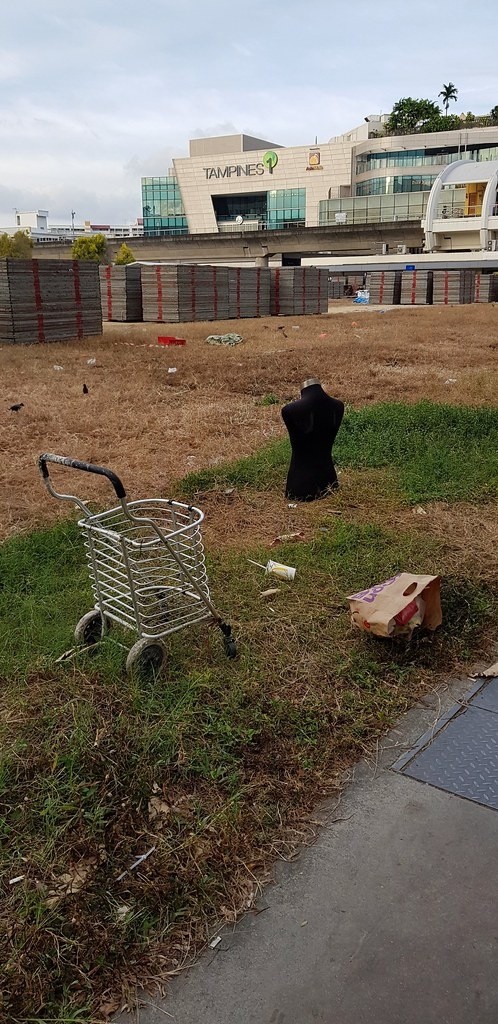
[37,452,238,682]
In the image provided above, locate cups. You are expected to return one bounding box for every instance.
[265,560,296,581]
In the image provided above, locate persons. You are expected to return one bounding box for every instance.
[281,378,344,502]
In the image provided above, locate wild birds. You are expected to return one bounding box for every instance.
[492,304,494,307]
[8,402,24,413]
[263,326,287,337]
[82,384,88,394]
